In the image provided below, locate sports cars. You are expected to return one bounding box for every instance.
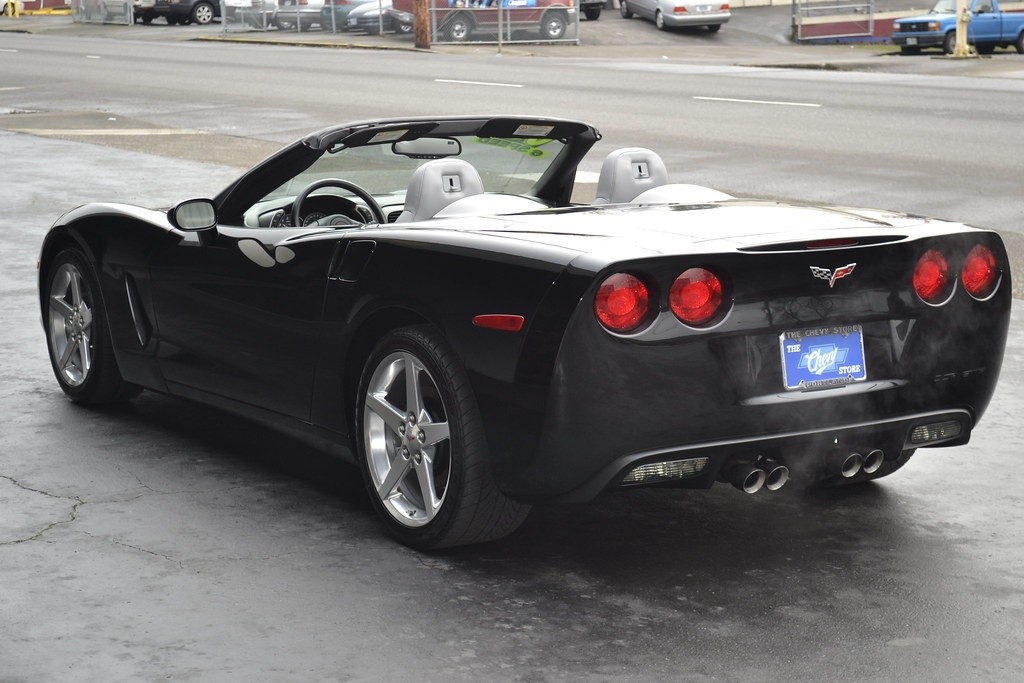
[38,114,1010,554]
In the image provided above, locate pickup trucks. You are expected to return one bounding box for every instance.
[891,0,1024,54]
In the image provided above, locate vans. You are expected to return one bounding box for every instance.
[388,1,575,41]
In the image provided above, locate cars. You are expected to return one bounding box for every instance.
[0,0,23,16]
[98,0,374,31]
[620,0,732,32]
[345,1,413,34]
[579,1,607,22]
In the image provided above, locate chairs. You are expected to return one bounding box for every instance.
[597,147,669,203]
[393,159,484,225]
